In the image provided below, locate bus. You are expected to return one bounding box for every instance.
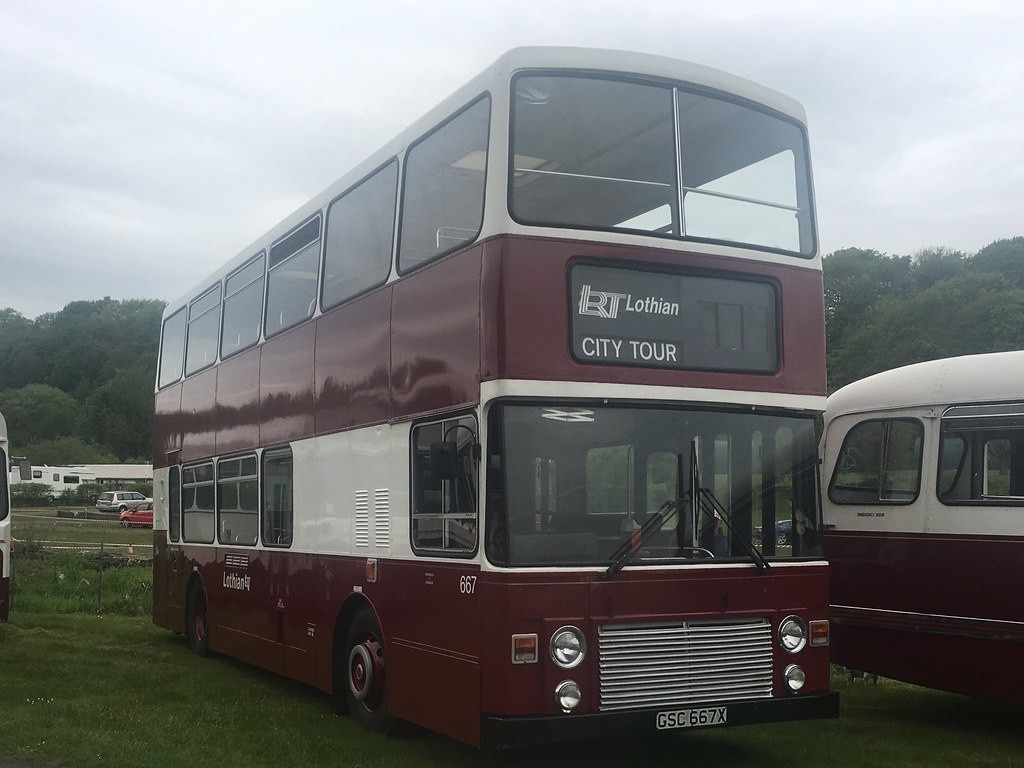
[151,46,839,756]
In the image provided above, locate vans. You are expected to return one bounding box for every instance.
[808,351,1024,708]
[119,501,153,528]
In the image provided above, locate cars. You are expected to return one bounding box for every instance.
[752,519,794,546]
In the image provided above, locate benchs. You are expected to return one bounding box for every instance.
[556,510,662,553]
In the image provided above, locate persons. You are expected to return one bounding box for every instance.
[698,508,729,556]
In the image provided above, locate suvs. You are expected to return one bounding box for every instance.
[95,490,154,512]
